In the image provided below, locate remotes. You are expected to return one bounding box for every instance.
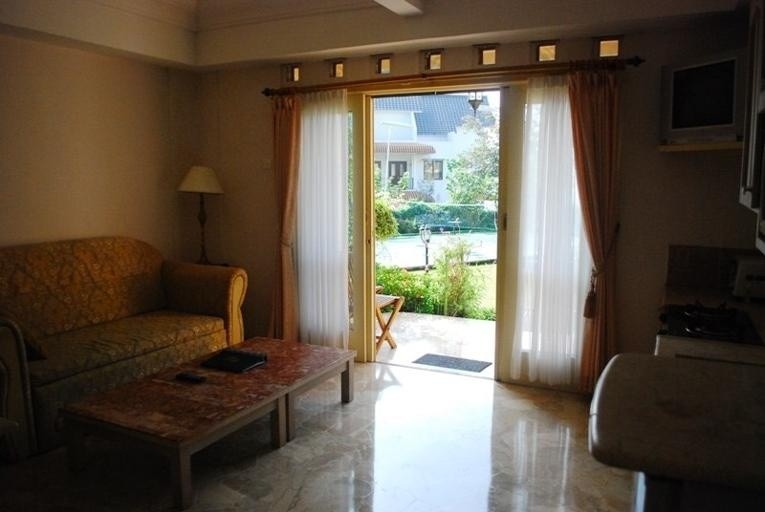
[174,372,207,384]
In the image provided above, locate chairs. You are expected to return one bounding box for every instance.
[347,252,405,357]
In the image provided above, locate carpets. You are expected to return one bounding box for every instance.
[412,353,492,372]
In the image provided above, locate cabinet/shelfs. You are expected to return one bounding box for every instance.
[738,0,765,256]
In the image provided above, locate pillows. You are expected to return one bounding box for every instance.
[0,306,49,359]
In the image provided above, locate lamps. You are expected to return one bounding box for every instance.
[176,166,225,265]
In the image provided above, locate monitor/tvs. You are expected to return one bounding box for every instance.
[659,46,746,144]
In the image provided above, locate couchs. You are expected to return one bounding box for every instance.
[0,236,248,464]
